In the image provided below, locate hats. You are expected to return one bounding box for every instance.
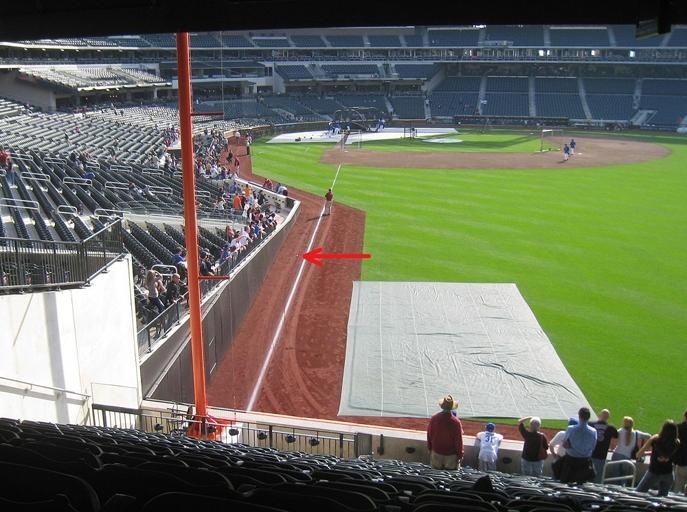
[487,423,495,432]
[569,417,579,425]
[438,394,458,410]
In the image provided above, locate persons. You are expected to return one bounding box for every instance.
[517,417,549,475]
[611,415,650,487]
[70,150,77,165]
[335,120,340,135]
[345,118,350,132]
[5,157,15,185]
[410,126,415,139]
[548,418,578,479]
[63,132,70,148]
[562,406,598,482]
[569,139,575,155]
[671,410,686,492]
[378,119,384,130]
[633,418,680,496]
[324,123,334,139]
[322,188,333,216]
[0,148,7,168]
[563,143,568,161]
[475,422,503,471]
[586,408,618,482]
[425,395,463,470]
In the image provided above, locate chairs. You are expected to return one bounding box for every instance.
[1,17,685,511]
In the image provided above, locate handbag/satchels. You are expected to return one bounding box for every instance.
[537,433,546,459]
[631,432,639,459]
